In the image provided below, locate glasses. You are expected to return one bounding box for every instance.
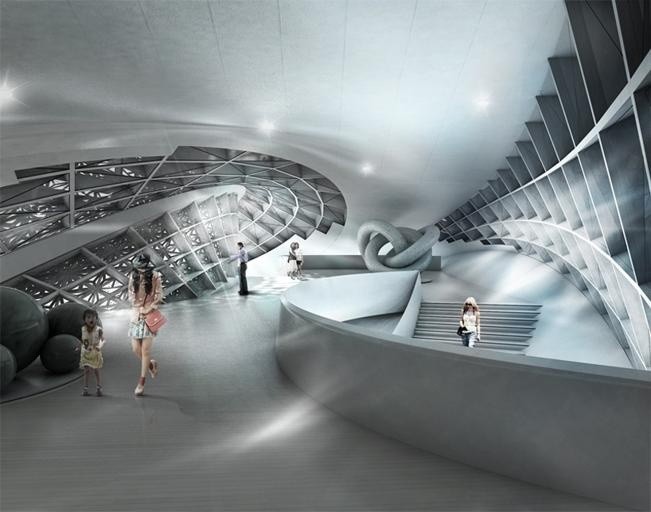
[466,303,472,306]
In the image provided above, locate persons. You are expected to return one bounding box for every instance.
[460,296,481,348]
[127,252,164,396]
[227,241,250,296]
[294,242,304,280]
[287,242,299,280]
[74,308,107,396]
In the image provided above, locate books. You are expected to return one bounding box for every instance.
[463,331,473,334]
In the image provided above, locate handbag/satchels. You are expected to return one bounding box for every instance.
[457,327,466,336]
[144,309,166,332]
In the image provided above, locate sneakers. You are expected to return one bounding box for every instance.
[135,384,145,395]
[96,385,102,394]
[150,360,158,378]
[81,385,88,396]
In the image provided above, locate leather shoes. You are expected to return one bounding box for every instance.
[238,290,248,295]
